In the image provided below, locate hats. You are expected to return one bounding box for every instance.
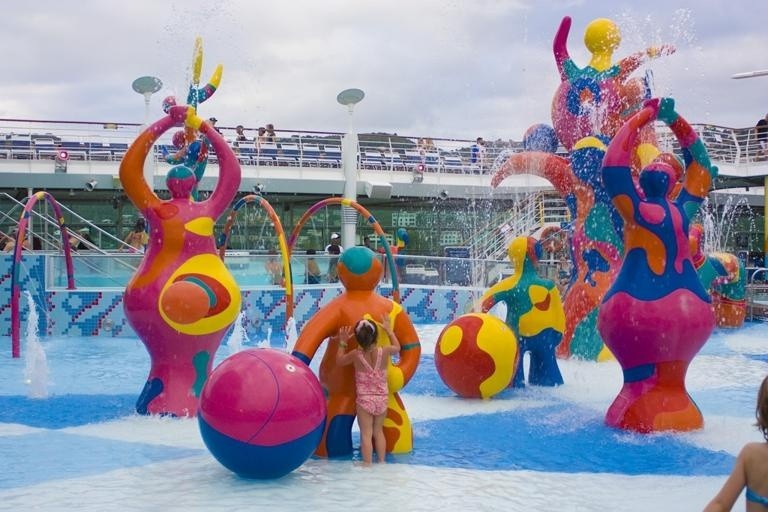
[331,234,339,240]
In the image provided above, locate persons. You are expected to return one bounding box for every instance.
[335,314,402,466]
[263,232,382,284]
[417,136,491,174]
[204,117,278,160]
[703,379,767,512]
[753,112,768,163]
[0,217,150,254]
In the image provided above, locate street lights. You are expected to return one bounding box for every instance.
[336,89,365,247]
[132,77,161,194]
